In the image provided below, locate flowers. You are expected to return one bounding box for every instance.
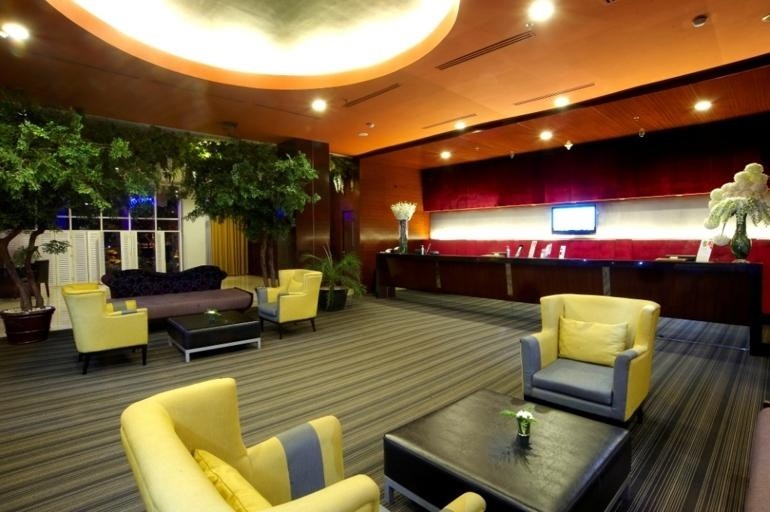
[390,200,417,221]
[500,403,538,434]
[704,162,770,247]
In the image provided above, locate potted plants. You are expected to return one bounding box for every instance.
[0,107,133,344]
[301,243,367,312]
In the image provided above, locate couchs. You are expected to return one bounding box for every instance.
[100,265,253,319]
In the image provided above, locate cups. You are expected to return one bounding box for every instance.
[516,410,532,436]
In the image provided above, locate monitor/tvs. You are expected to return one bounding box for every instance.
[551,203,598,236]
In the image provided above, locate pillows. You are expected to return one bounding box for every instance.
[287,279,304,292]
[558,315,627,367]
[194,449,273,512]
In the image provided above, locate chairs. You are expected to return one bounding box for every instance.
[254,269,323,339]
[120,377,487,512]
[519,294,661,423]
[61,282,148,375]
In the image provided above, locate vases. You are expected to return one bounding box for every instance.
[399,220,408,254]
[730,212,752,259]
[517,418,531,437]
